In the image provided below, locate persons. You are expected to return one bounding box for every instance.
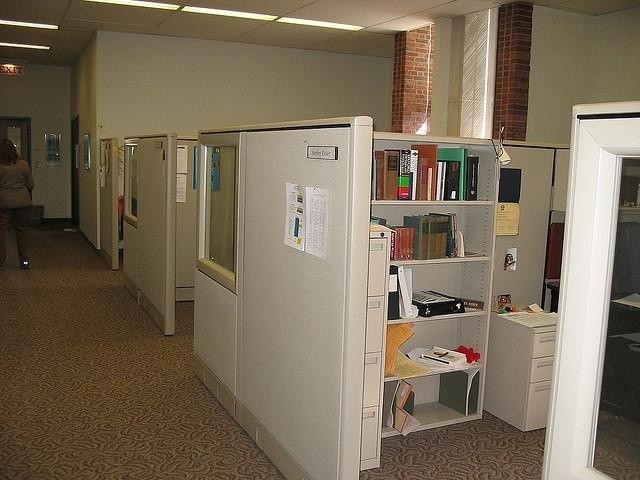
[0,139,34,270]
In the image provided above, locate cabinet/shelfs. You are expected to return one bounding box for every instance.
[481,305,558,434]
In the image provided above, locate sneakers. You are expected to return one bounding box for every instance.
[20,257,30,269]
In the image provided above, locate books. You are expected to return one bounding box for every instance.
[382,380,422,437]
[372,144,479,202]
[386,322,469,379]
[372,211,465,259]
